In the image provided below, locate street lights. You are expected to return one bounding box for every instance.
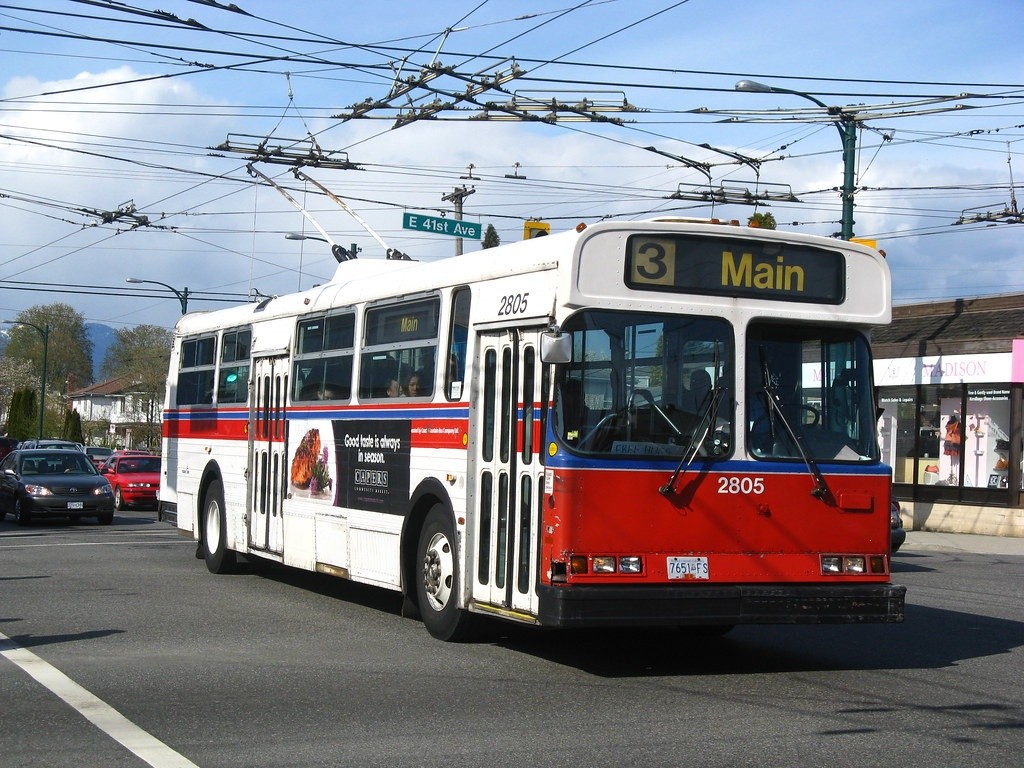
[735,79,857,240]
[2,319,49,440]
[126,277,189,315]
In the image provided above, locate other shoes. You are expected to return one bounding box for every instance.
[996,454,1009,471]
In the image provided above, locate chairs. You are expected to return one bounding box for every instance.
[23,447,160,472]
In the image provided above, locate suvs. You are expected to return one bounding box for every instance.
[0,436,17,463]
[21,439,84,470]
[81,445,113,469]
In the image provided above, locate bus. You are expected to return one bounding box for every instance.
[155,160,908,645]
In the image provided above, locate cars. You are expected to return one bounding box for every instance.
[98,450,151,471]
[99,455,162,511]
[0,449,115,527]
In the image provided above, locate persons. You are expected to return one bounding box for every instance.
[300,347,434,397]
[63,458,80,473]
[944,414,961,456]
[559,379,592,448]
[682,369,730,418]
[21,460,41,473]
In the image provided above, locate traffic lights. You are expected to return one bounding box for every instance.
[523,221,551,240]
